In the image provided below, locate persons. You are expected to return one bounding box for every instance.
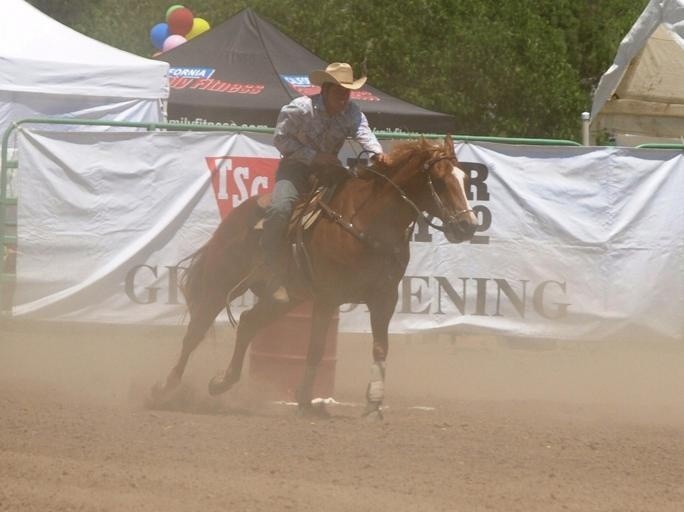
[257,62,393,306]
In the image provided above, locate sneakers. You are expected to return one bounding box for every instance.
[272,286,290,305]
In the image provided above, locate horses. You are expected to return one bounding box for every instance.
[149,130,477,424]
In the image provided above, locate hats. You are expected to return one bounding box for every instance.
[309,62,367,90]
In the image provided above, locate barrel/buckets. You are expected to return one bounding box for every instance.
[250,291,340,402]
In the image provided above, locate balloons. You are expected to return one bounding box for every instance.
[148,3,210,61]
